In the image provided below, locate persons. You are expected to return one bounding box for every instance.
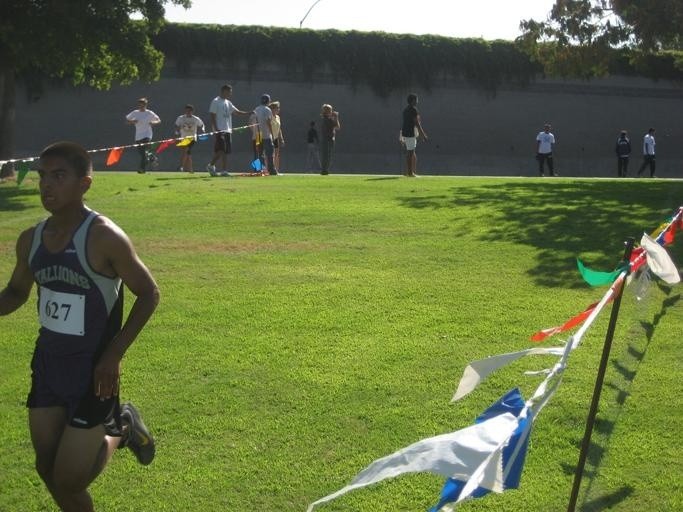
[399,93,427,176]
[1,140,160,512]
[616,130,631,177]
[535,123,558,177]
[306,121,322,168]
[319,103,341,175]
[637,127,658,177]
[125,84,286,177]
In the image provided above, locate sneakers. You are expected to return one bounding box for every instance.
[206,163,218,177]
[407,169,419,177]
[251,166,279,176]
[220,171,231,177]
[116,399,156,466]
[137,167,146,173]
[320,169,329,175]
[147,152,158,163]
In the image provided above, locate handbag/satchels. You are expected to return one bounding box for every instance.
[255,129,263,148]
[400,126,420,143]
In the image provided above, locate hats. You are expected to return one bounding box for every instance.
[261,94,272,104]
